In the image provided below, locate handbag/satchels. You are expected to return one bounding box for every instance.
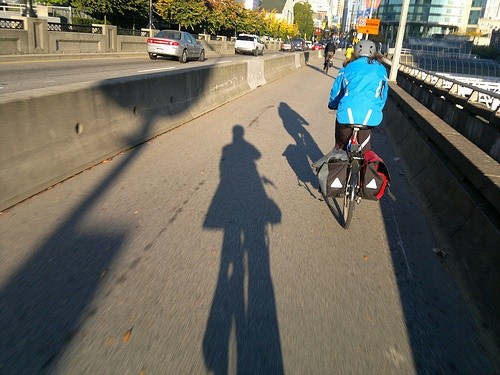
[360,150,392,200]
[330,61,333,67]
[311,148,349,198]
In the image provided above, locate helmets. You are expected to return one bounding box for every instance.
[354,40,376,57]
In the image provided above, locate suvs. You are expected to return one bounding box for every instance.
[234,33,265,56]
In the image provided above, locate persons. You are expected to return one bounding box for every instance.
[328,40,388,156]
[325,41,335,60]
[344,44,355,59]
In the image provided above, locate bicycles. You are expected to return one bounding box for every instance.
[324,52,335,75]
[328,104,384,229]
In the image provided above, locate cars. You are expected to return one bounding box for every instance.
[281,38,331,52]
[146,30,205,63]
